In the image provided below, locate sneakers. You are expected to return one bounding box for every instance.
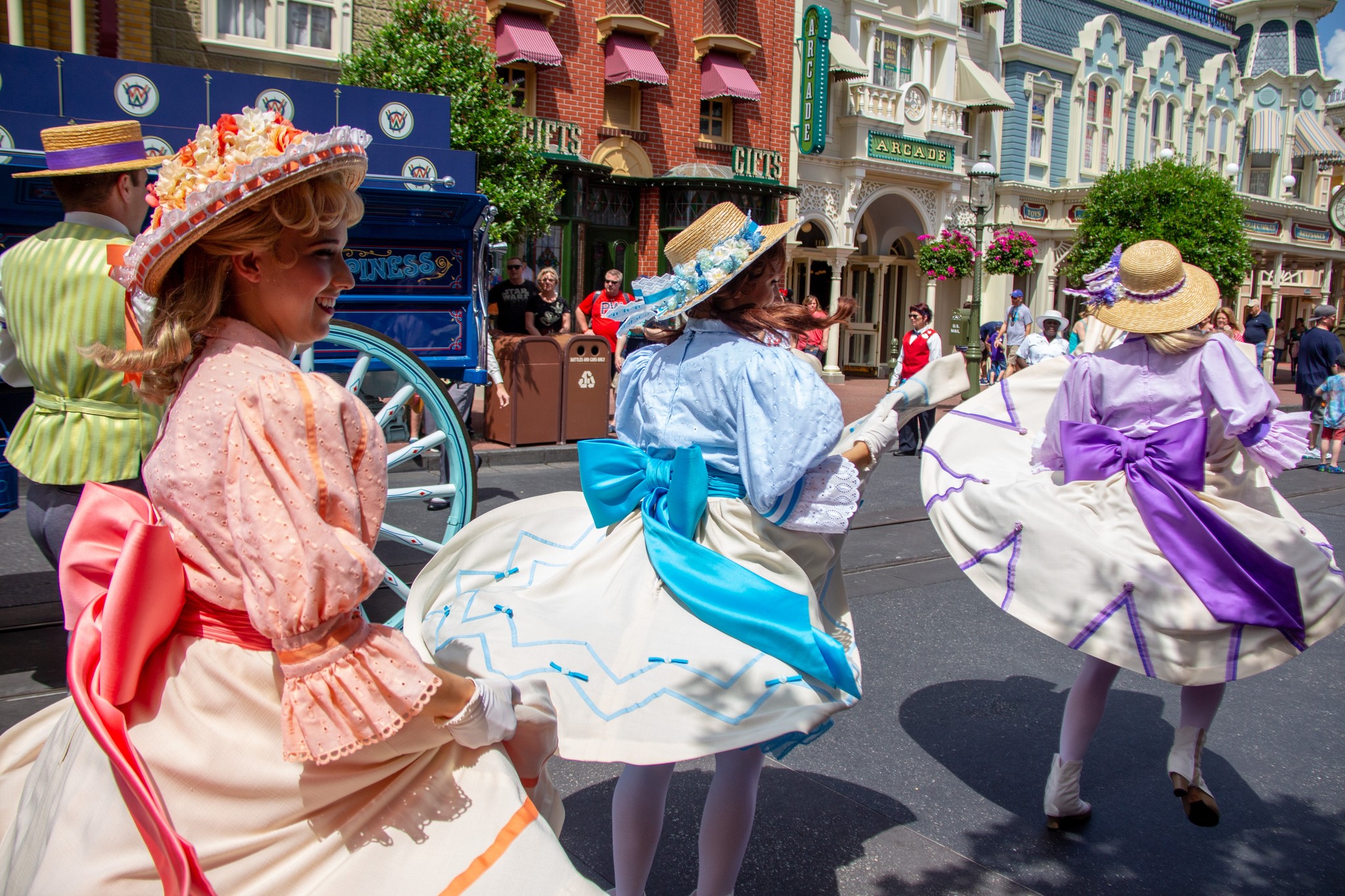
[1303,445,1333,460]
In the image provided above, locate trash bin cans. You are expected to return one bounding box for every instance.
[547,330,611,444]
[484,333,563,447]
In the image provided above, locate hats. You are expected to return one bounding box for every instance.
[1246,299,1260,307]
[642,197,809,325]
[1308,306,1336,322]
[118,102,372,301]
[1036,310,1070,332]
[14,119,176,177]
[1010,289,1024,298]
[994,321,1003,330]
[1085,239,1220,332]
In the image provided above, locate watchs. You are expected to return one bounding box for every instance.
[1265,344,1270,348]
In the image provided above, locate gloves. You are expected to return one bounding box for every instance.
[854,408,900,469]
[434,677,521,750]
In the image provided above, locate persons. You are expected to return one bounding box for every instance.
[1296,304,1345,474]
[482,251,688,440]
[979,289,1131,388]
[1044,240,1314,830]
[1197,299,1274,382]
[1289,318,1307,381]
[406,392,425,444]
[1272,318,1287,381]
[615,201,898,896]
[419,283,511,511]
[886,302,944,460]
[740,294,829,366]
[0,118,175,571]
[140,107,515,896]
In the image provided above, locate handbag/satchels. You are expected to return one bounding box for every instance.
[804,342,820,359]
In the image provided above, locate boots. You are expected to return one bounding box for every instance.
[1042,754,1093,829]
[1165,723,1219,827]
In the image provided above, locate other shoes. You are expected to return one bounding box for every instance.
[1317,463,1327,472]
[979,378,990,386]
[1328,464,1345,474]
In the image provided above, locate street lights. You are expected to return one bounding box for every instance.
[961,147,1014,404]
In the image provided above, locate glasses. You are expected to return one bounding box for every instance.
[908,313,922,320]
[507,264,523,270]
[1010,295,1018,300]
[605,280,620,285]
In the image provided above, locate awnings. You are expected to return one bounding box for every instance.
[1290,111,1345,171]
[660,163,802,200]
[534,152,611,177]
[700,53,762,105]
[604,36,669,91]
[955,56,1015,114]
[960,0,1008,18]
[1245,109,1282,157]
[827,32,869,82]
[496,14,562,74]
